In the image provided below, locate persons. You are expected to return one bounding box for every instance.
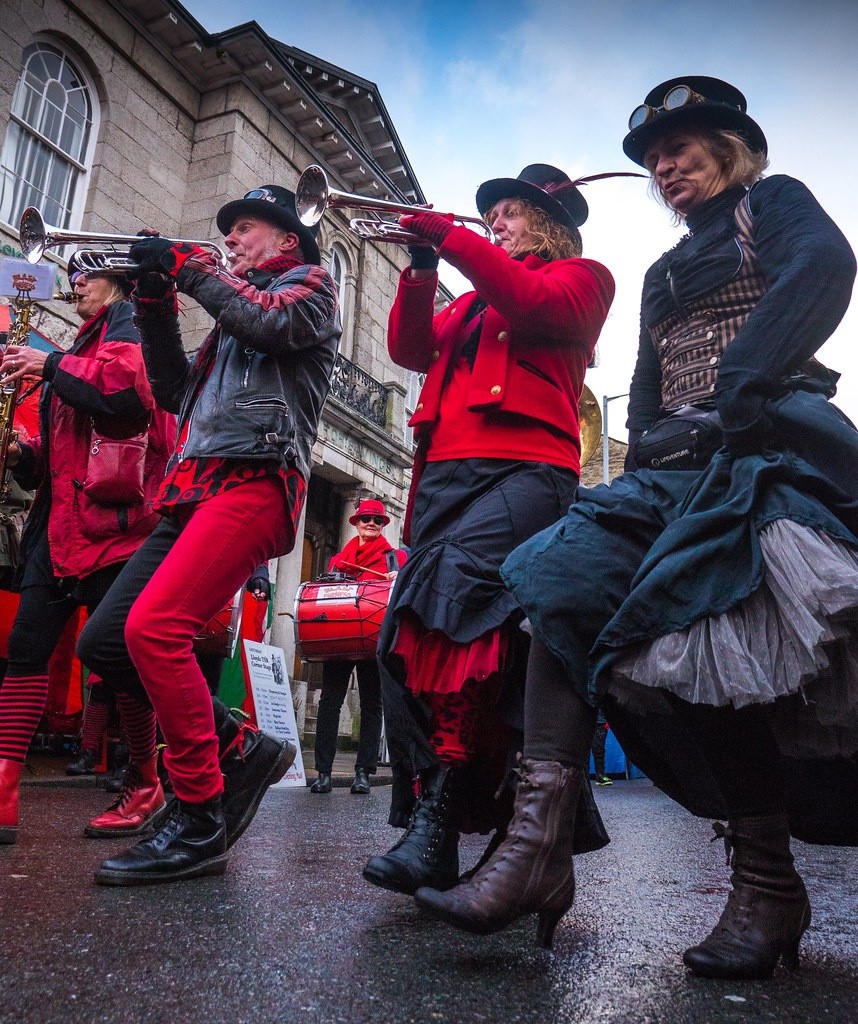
[412,76,858,977]
[66,559,271,788]
[0,247,181,846]
[593,707,614,786]
[310,500,409,794]
[359,162,611,893]
[94,185,342,884]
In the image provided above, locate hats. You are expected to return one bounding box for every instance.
[216,184,321,266]
[475,164,650,257]
[622,76,769,168]
[69,248,140,299]
[349,497,389,526]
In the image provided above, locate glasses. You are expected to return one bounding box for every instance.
[243,189,277,205]
[67,267,103,284]
[628,85,742,131]
[357,515,386,526]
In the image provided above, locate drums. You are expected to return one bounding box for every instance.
[293,575,400,663]
[190,583,246,662]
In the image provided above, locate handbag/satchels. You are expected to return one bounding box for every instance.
[632,404,724,472]
[85,424,148,506]
[0,508,31,569]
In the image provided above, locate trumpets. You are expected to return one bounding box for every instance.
[292,161,504,251]
[17,205,238,277]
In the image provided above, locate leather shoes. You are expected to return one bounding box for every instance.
[350,766,370,793]
[311,771,332,793]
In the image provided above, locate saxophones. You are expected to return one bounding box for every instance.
[0,291,84,497]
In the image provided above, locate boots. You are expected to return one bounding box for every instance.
[362,763,460,896]
[84,750,167,837]
[683,811,812,981]
[0,758,25,844]
[64,749,98,774]
[460,774,515,885]
[414,751,575,950]
[214,699,297,851]
[107,743,132,794]
[94,798,228,885]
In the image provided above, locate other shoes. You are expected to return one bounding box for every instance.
[595,773,613,785]
